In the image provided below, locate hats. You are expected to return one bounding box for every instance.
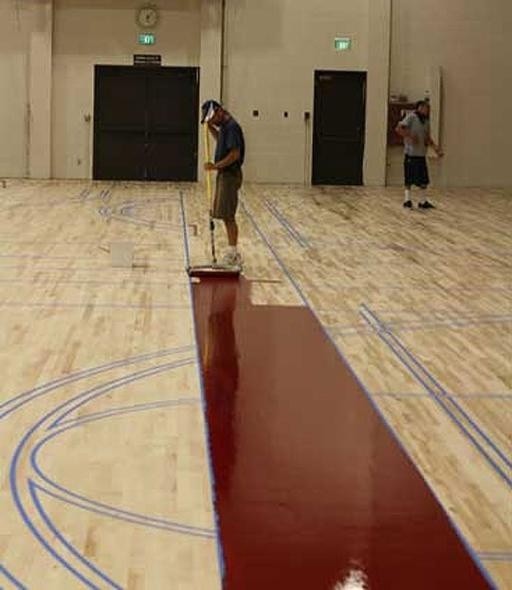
[200,100,219,124]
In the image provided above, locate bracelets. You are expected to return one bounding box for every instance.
[215,162,222,168]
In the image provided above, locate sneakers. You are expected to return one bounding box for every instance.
[404,200,437,210]
[222,253,244,266]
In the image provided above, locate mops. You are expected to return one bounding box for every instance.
[185,120,243,278]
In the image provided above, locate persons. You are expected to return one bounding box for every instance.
[394,100,444,209]
[200,100,245,266]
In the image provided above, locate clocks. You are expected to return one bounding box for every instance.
[135,5,161,29]
